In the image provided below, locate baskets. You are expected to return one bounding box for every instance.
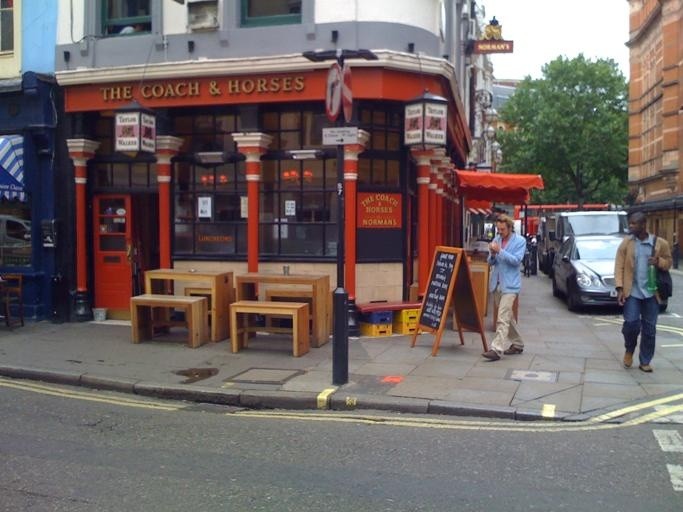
[358,308,423,336]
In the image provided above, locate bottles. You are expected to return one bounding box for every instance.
[648,264,658,293]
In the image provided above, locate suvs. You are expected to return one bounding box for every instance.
[536,218,554,270]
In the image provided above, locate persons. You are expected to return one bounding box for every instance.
[481,214,527,360]
[615,211,672,372]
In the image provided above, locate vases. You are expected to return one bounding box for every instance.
[92,308,106,321]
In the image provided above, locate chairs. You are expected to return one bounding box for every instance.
[0,274,25,326]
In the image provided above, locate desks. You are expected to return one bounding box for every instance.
[144,268,234,342]
[236,274,330,348]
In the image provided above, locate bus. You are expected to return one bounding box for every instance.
[514,204,610,234]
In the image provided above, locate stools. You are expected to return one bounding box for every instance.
[266,288,334,336]
[130,294,210,348]
[229,300,310,357]
[183,287,235,329]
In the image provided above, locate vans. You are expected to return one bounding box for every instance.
[554,211,638,246]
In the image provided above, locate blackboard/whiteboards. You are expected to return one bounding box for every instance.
[415,246,462,334]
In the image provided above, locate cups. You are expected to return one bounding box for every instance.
[283,265,290,276]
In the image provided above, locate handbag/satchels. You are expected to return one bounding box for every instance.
[656,265,672,300]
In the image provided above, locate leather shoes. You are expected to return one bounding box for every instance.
[623,351,633,366]
[639,363,653,372]
[503,346,522,354]
[482,350,500,360]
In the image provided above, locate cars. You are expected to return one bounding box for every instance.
[552,236,670,312]
